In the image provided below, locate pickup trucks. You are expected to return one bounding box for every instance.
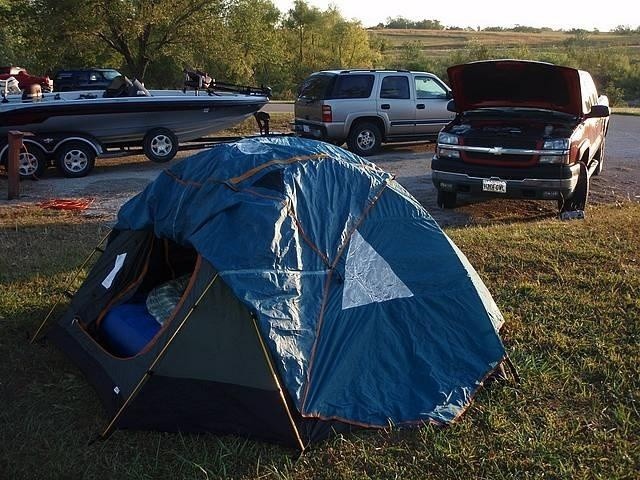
[428,58,614,217]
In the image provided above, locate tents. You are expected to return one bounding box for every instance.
[27,133,521,460]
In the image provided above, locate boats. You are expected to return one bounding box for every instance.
[1,72,274,147]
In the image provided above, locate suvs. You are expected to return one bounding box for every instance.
[0,64,57,93]
[56,67,122,90]
[290,67,458,159]
